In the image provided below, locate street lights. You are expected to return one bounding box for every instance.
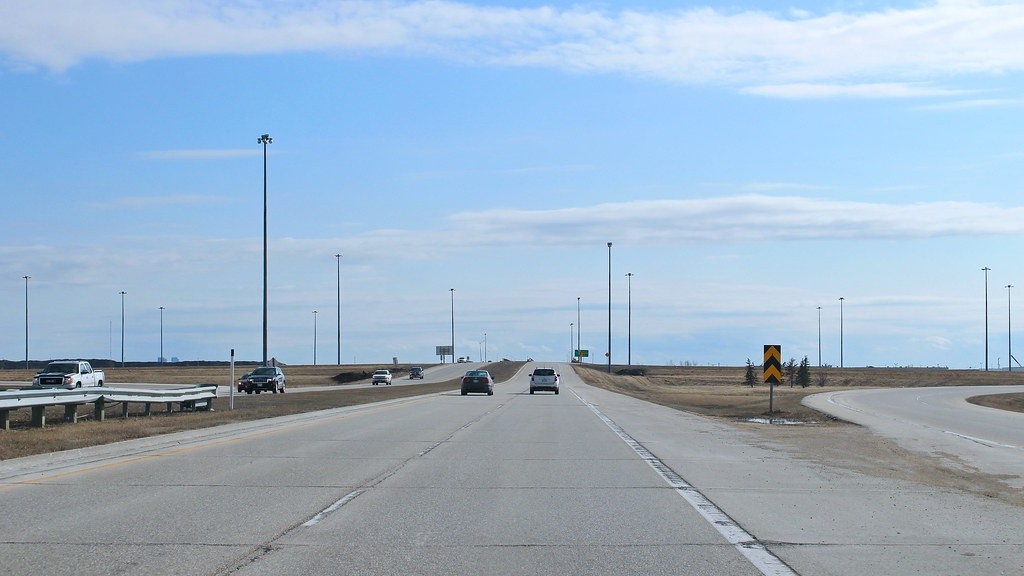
[607,241,613,373]
[816,306,823,368]
[571,295,581,363]
[982,267,991,371]
[336,254,342,363]
[22,276,32,369]
[158,305,164,365]
[120,292,127,366]
[259,134,271,360]
[312,310,317,364]
[450,289,456,362]
[839,297,846,367]
[626,273,633,365]
[1005,284,1014,371]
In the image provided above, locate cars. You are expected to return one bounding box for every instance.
[461,370,495,396]
[458,357,466,363]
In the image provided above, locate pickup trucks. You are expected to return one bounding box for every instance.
[33,360,105,388]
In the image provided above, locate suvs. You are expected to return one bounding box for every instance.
[238,373,253,392]
[528,368,561,396]
[409,367,424,379]
[246,367,286,394]
[371,370,392,385]
